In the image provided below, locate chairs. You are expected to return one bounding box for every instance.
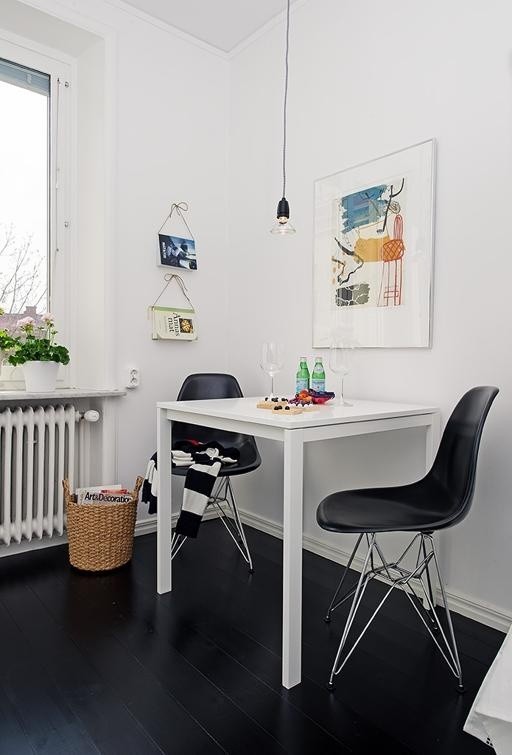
[151,371,264,574]
[313,385,500,696]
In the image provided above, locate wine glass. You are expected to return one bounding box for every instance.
[258,340,283,398]
[328,339,351,407]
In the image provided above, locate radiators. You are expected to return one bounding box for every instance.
[0,403,91,549]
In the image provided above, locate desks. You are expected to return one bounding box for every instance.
[154,399,444,690]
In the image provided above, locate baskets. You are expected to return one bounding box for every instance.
[62,474,144,572]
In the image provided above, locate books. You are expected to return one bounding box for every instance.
[157,232,198,273]
[75,485,134,506]
[152,306,198,342]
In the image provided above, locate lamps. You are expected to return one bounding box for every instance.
[269,3,297,237]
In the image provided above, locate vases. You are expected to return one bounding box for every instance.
[23,358,57,394]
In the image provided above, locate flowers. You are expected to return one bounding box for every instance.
[0,308,70,365]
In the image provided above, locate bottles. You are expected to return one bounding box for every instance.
[296,357,326,393]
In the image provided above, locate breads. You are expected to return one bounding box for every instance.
[257,401,288,409]
[272,408,303,415]
